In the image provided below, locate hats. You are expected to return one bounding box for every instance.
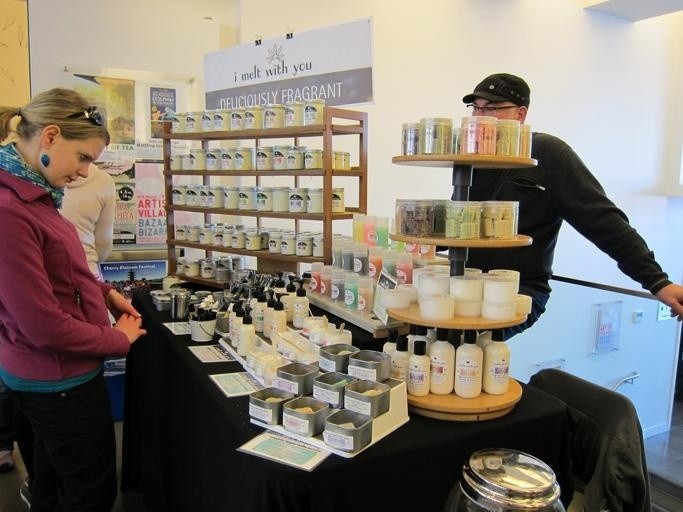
[462,73,530,109]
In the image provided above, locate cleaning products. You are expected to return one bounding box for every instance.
[483,330,509,393]
[384,326,454,396]
[454,332,483,399]
[228,271,310,357]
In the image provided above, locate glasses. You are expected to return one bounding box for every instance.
[65,103,108,130]
[466,104,516,115]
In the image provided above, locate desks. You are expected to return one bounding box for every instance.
[130,287,597,512]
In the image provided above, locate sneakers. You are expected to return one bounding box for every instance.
[0,447,15,474]
[20,475,32,508]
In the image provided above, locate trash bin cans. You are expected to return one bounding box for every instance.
[102,358,126,422]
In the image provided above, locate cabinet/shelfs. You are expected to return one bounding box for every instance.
[151,105,367,301]
[386,150,540,425]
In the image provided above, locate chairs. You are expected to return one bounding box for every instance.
[524,364,653,512]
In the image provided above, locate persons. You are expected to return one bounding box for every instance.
[412,73,683,361]
[109,115,136,144]
[0,382,18,475]
[54,160,118,286]
[0,86,148,511]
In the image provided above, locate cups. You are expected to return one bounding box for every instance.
[244,314,354,384]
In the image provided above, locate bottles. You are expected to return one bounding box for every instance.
[397,114,536,158]
[383,322,511,397]
[306,213,437,315]
[170,290,190,321]
[395,198,519,239]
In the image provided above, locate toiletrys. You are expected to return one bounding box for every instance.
[173,244,186,276]
[379,323,511,401]
[226,270,311,357]
